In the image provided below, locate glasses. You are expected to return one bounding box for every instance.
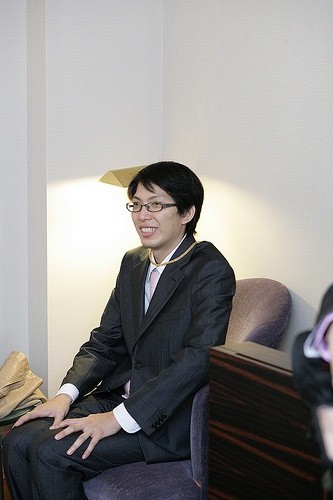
[126,202,178,212]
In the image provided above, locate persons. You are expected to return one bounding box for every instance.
[1,160,236,499]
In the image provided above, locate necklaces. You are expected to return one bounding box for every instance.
[147,240,199,267]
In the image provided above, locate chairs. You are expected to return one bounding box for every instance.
[80,279,290,500]
